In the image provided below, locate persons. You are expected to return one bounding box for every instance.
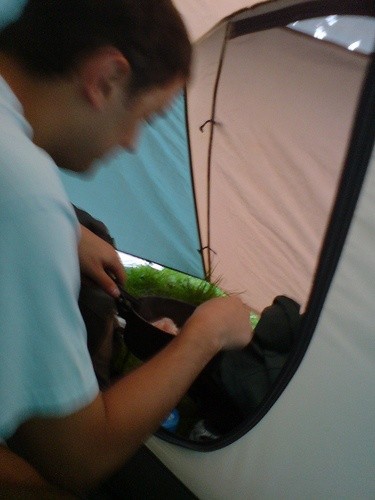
[0,0,255,500]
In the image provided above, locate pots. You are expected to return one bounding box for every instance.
[101,281,198,362]
